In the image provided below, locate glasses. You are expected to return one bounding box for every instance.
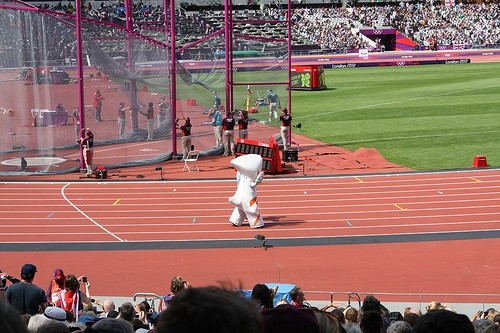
[295,293,306,297]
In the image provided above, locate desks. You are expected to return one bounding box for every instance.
[261,282,296,304]
[30,108,69,127]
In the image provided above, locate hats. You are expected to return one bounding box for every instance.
[225,112,231,117]
[212,104,219,108]
[281,107,288,113]
[145,285,262,333]
[267,89,273,93]
[258,303,340,333]
[27,306,87,333]
[20,263,37,274]
[411,309,475,333]
[86,130,91,136]
[361,295,381,313]
[241,110,248,115]
[185,117,190,123]
[389,311,403,321]
[52,269,63,280]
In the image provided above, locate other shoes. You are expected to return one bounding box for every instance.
[223,152,228,157]
[232,152,235,156]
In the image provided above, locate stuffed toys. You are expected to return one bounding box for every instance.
[228,154,264,229]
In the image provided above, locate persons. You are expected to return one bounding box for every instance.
[73,108,81,143]
[157,97,169,127]
[236,111,248,139]
[118,102,131,137]
[93,89,104,122]
[221,113,235,156]
[0,0,500,69]
[264,89,280,122]
[81,129,94,175]
[209,92,223,149]
[139,101,153,141]
[0,269,500,333]
[6,264,48,316]
[179,119,192,159]
[280,108,291,150]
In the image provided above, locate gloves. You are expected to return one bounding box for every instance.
[255,170,264,184]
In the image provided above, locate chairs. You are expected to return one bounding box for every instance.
[181,150,200,173]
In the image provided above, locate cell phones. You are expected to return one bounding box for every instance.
[83,277,87,283]
